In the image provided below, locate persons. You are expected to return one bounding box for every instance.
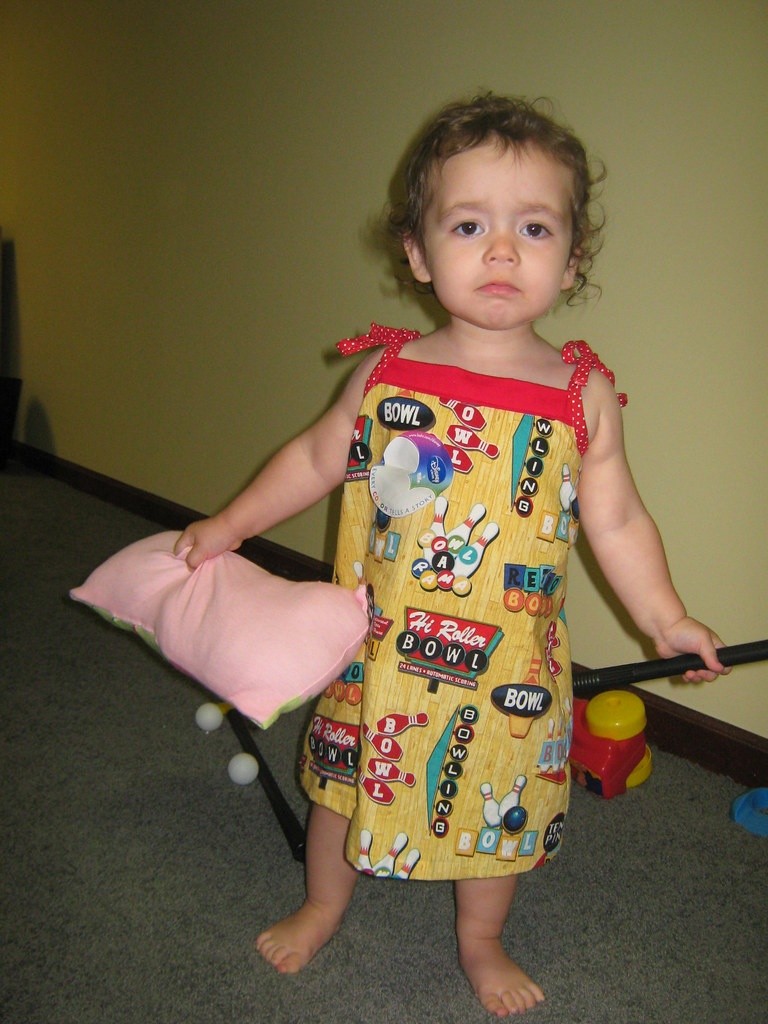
[172,90,734,1017]
